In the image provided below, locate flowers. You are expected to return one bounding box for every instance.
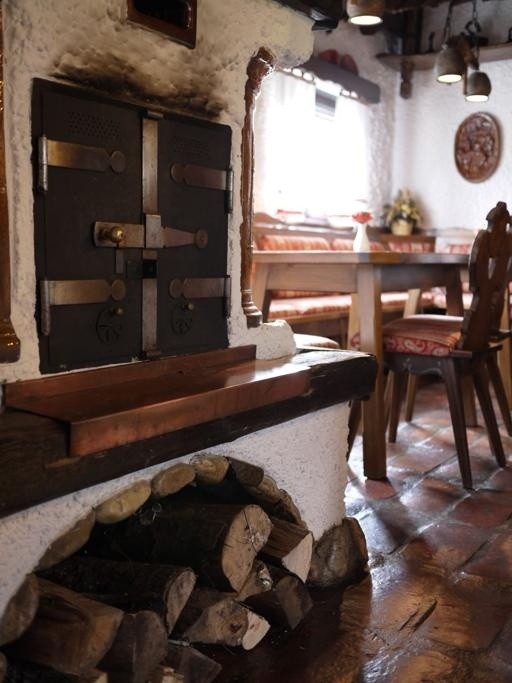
[380,191,423,228]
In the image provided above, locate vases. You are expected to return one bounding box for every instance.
[388,215,415,236]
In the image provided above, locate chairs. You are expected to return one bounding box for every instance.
[348,200,510,488]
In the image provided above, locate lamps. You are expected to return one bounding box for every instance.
[434,0,490,103]
[343,0,386,27]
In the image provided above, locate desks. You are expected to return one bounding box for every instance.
[247,247,471,480]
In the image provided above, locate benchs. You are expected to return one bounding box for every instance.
[244,232,512,347]
